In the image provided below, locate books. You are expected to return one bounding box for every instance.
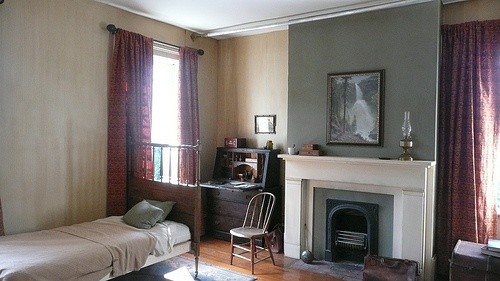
[481,239,500,257]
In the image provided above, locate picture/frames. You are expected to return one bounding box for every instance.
[326,69,385,147]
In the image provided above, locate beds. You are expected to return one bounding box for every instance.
[0,136,200,281]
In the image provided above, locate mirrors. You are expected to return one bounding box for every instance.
[255,115,276,134]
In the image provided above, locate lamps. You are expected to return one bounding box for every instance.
[398,112,413,161]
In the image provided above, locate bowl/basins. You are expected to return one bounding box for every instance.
[288,147,298,155]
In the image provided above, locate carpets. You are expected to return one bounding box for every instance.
[110,256,257,281]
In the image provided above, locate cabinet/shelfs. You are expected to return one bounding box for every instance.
[199,147,281,247]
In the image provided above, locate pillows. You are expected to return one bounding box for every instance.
[146,199,176,223]
[121,199,164,229]
[165,209,177,221]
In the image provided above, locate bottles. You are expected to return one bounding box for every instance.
[266,140,273,149]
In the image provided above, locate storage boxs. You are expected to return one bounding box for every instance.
[224,137,246,148]
[449,239,500,281]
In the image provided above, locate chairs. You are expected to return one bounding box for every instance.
[229,192,276,275]
